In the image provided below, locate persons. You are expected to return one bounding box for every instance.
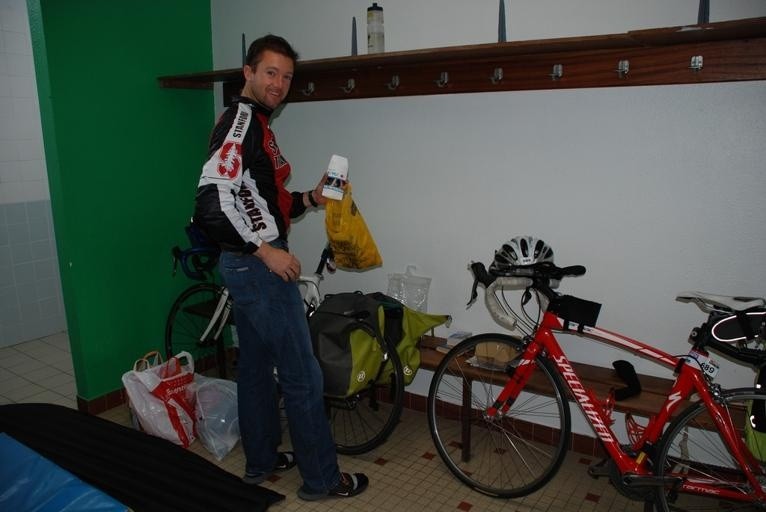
[194,34,369,501]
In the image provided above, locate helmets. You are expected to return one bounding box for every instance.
[494,236,553,269]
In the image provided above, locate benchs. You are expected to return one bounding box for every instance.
[182,292,748,463]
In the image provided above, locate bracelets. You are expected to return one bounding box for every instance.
[308,190,318,208]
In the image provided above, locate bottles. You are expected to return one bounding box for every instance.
[366,4,384,52]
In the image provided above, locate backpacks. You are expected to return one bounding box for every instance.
[307,291,451,400]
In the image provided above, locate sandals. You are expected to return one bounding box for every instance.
[242,451,296,485]
[296,472,368,501]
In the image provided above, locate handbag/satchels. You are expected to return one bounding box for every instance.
[745,364,765,460]
[121,351,198,448]
[325,183,383,271]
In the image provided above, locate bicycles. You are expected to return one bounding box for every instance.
[427,262,766,512]
[164,241,406,457]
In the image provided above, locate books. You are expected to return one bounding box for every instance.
[436,331,474,358]
